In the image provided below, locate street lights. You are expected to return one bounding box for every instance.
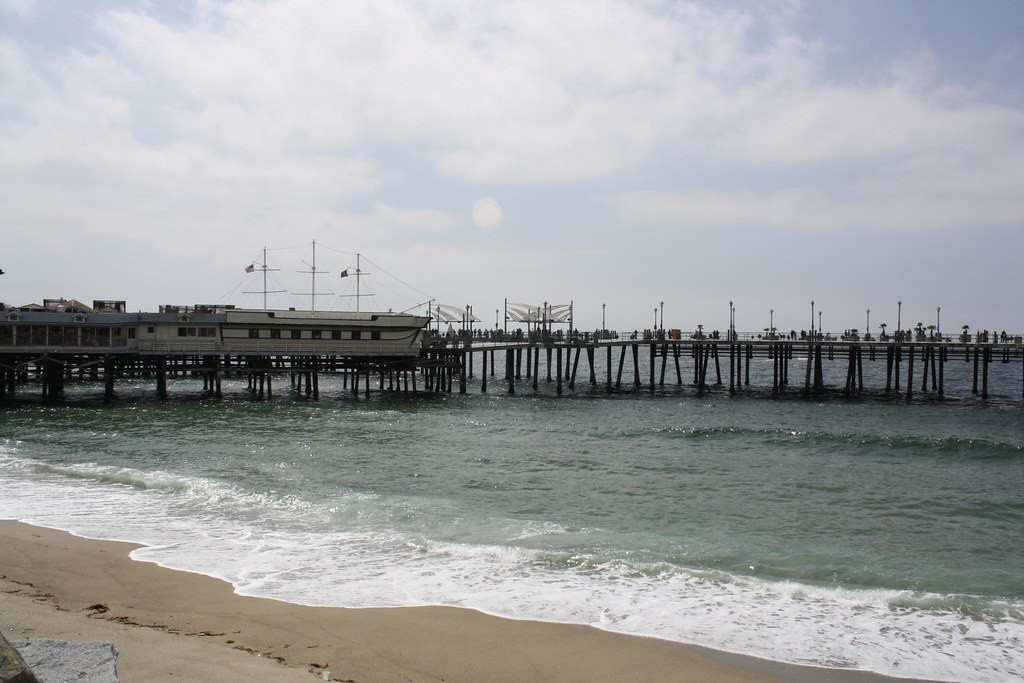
[811,300,814,341]
[544,300,548,331]
[602,303,606,338]
[537,305,541,333]
[866,309,870,334]
[732,306,735,339]
[436,306,440,335]
[729,299,733,341]
[897,300,902,332]
[661,301,664,336]
[466,303,470,332]
[654,307,658,337]
[937,305,941,334]
[770,309,773,338]
[818,310,822,334]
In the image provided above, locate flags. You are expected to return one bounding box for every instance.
[245,264,254,273]
[341,270,348,277]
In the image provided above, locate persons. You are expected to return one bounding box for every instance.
[713,330,719,339]
[511,328,523,339]
[984,329,1006,341]
[432,327,493,338]
[844,330,850,340]
[790,329,804,340]
